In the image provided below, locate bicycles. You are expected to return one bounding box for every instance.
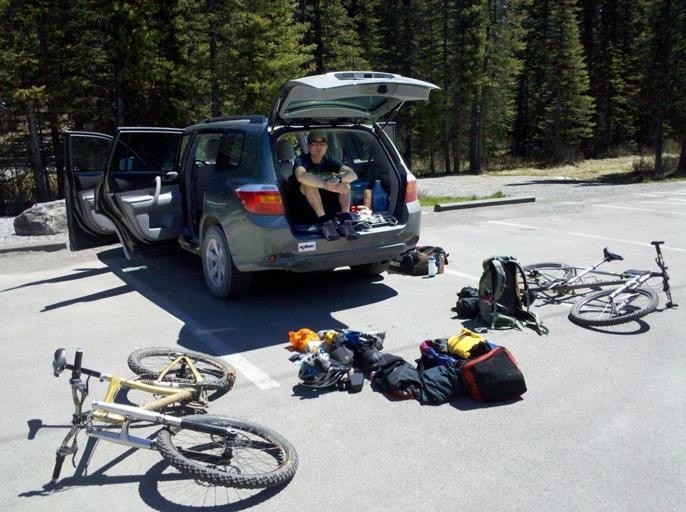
[52,348,298,490]
[517,241,679,327]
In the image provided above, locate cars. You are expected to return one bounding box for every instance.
[63,70,441,300]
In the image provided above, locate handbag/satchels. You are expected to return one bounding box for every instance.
[419,328,527,404]
[402,245,447,275]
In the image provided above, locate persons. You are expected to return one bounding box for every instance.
[290,130,359,241]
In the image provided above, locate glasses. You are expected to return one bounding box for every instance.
[311,139,326,145]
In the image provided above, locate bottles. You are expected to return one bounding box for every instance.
[363,189,372,209]
[428,251,445,277]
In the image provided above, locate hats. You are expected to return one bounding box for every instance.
[306,129,328,142]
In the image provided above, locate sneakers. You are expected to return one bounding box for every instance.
[322,219,340,241]
[339,220,358,241]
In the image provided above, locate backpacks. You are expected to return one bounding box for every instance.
[478,256,549,336]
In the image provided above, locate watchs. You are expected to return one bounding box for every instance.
[336,174,342,183]
